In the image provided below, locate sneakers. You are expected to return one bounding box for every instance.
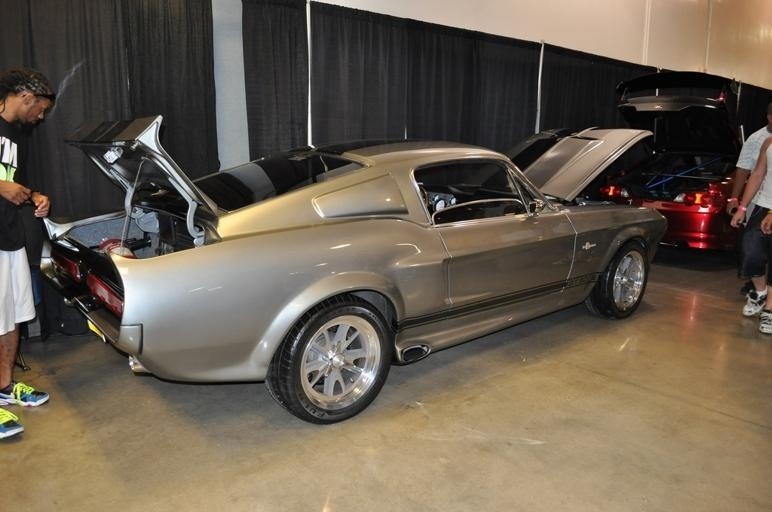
[0,408,24,439]
[758,310,772,333]
[742,289,767,316]
[742,280,756,295]
[0,381,50,406]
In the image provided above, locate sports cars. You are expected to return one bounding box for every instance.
[39,115,668,425]
[599,71,752,252]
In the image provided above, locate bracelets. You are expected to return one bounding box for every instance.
[739,205,747,212]
[767,211,772,213]
[727,198,738,203]
[29,190,41,198]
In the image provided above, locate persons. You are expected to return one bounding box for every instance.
[760,209,772,235]
[730,137,772,333]
[0,70,56,439]
[725,103,772,296]
